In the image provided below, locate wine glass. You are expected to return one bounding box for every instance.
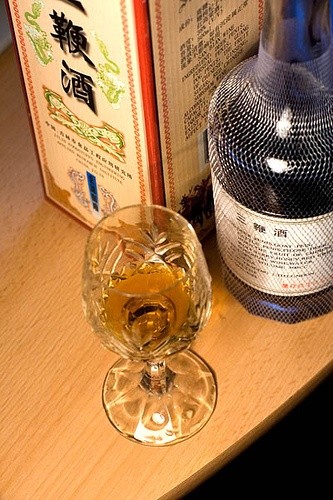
[80,204,217,447]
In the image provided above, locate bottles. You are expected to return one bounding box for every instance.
[205,0,332,323]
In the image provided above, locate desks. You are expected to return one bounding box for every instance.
[1,2,333,498]
